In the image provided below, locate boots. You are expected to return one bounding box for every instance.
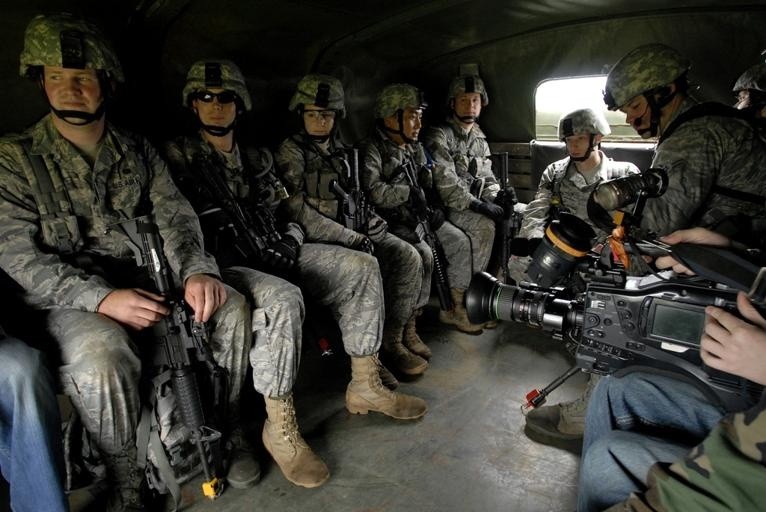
[344,287,498,421]
[262,391,331,488]
[525,370,603,441]
[99,447,144,511]
[210,404,263,490]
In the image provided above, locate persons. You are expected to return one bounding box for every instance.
[273,72,429,389]
[362,81,482,358]
[2,14,260,511]
[1,329,70,512]
[507,42,766,511]
[155,58,427,487]
[426,75,517,329]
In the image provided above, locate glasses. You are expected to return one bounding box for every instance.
[302,109,337,120]
[194,90,238,103]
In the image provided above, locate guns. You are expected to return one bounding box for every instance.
[199,150,285,269]
[328,146,369,233]
[402,160,454,310]
[493,151,517,272]
[107,213,224,499]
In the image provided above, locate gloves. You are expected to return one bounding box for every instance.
[263,236,300,272]
[469,199,504,219]
[351,232,374,255]
[411,186,427,208]
[368,211,388,243]
[493,186,518,207]
[430,208,444,230]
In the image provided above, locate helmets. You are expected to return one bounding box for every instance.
[602,43,694,111]
[732,67,765,92]
[17,12,125,84]
[373,83,428,120]
[557,108,611,142]
[447,73,489,107]
[181,58,252,111]
[287,74,347,120]
